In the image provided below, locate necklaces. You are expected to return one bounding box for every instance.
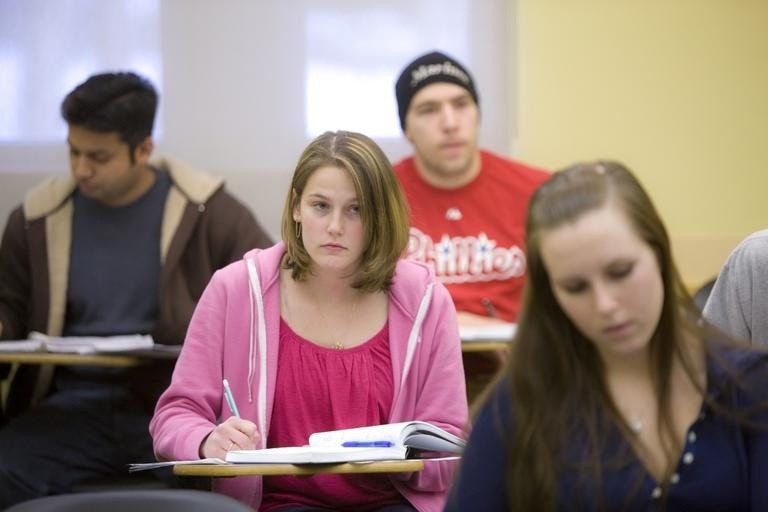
[628,419,643,434]
[307,285,359,351]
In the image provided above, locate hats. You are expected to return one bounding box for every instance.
[396,53,478,130]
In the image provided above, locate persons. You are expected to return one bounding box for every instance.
[392,52,554,323]
[147,131,474,512]
[0,72,274,512]
[443,160,768,512]
[702,229,768,351]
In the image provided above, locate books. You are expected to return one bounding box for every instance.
[225,420,470,464]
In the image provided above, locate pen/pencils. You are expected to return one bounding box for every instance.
[342,441,396,447]
[222,379,240,419]
